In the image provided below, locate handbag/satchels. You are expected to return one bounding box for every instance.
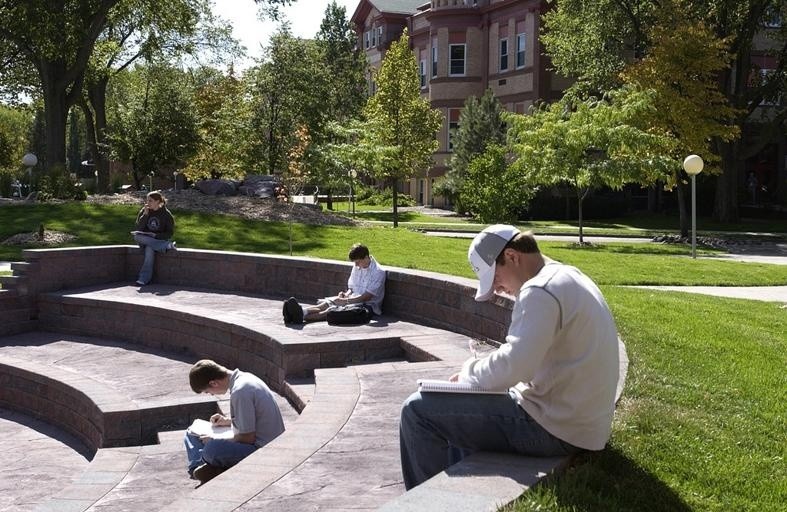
[327,304,370,324]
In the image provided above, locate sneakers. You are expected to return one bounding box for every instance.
[171,241,177,250]
[194,462,226,481]
[136,280,148,285]
[282,297,304,326]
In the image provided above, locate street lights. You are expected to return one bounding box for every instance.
[684,155,704,257]
[23,152,38,194]
[173,171,178,191]
[148,170,155,189]
[347,169,357,214]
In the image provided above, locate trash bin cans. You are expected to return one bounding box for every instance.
[81,159,99,194]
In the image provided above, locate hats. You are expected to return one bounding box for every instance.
[469,223,521,301]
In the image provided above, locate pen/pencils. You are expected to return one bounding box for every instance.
[216,416,224,422]
[344,288,351,296]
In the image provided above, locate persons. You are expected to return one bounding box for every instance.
[399,222,622,490]
[283,243,386,324]
[131,191,176,284]
[182,358,283,480]
